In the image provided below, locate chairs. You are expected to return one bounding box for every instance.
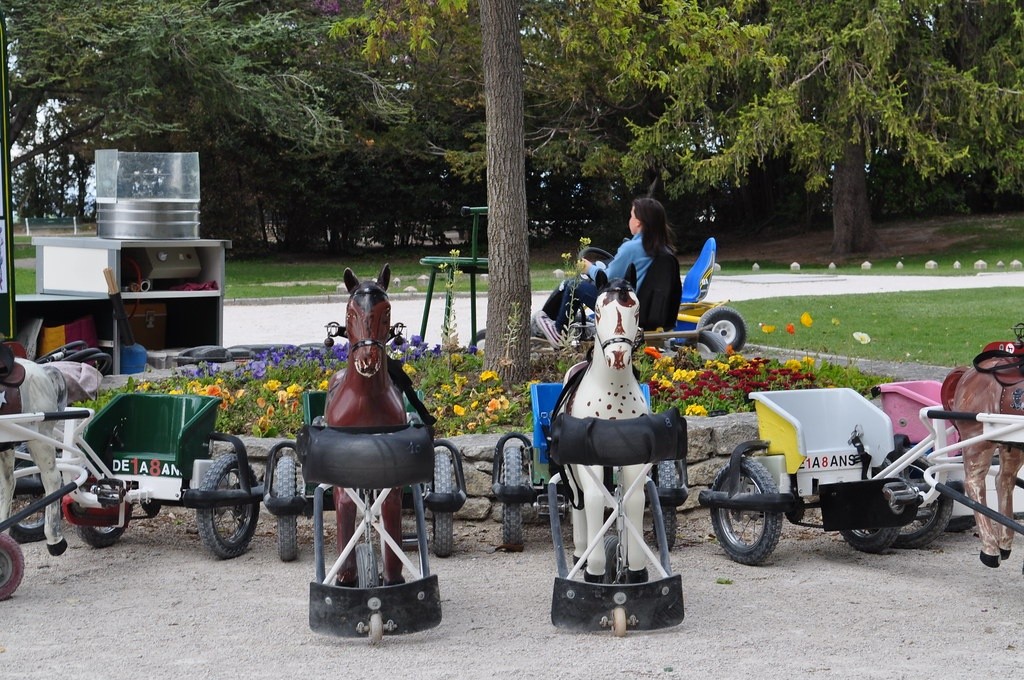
[636,254,682,329]
[681,237,716,309]
[301,386,424,426]
[85,391,224,479]
[530,382,651,462]
[750,387,896,488]
[419,206,489,346]
[877,378,961,456]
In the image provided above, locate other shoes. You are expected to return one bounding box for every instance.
[536,311,563,349]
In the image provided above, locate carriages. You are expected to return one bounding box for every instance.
[0,335,271,607]
[697,327,1024,570]
[257,260,464,645]
[483,268,697,635]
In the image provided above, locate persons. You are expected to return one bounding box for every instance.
[527,198,675,350]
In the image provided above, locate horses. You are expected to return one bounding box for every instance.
[320,264,412,589]
[546,263,654,587]
[940,356,1024,569]
[0,355,68,557]
